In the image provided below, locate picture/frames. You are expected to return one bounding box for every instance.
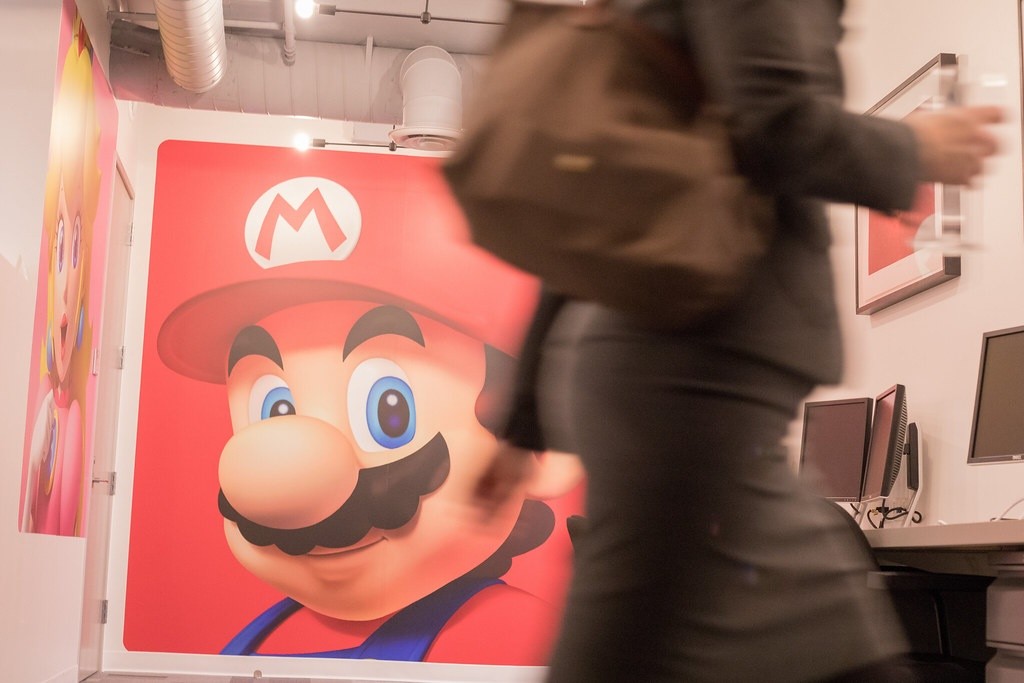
[853,53,962,316]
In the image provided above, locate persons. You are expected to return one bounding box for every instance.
[538,0,1006,683]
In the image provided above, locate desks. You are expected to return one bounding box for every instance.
[861,519,1024,683]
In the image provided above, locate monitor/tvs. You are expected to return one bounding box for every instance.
[967,325,1024,466]
[798,382,924,528]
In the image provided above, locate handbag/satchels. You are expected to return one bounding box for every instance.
[440,1,768,315]
